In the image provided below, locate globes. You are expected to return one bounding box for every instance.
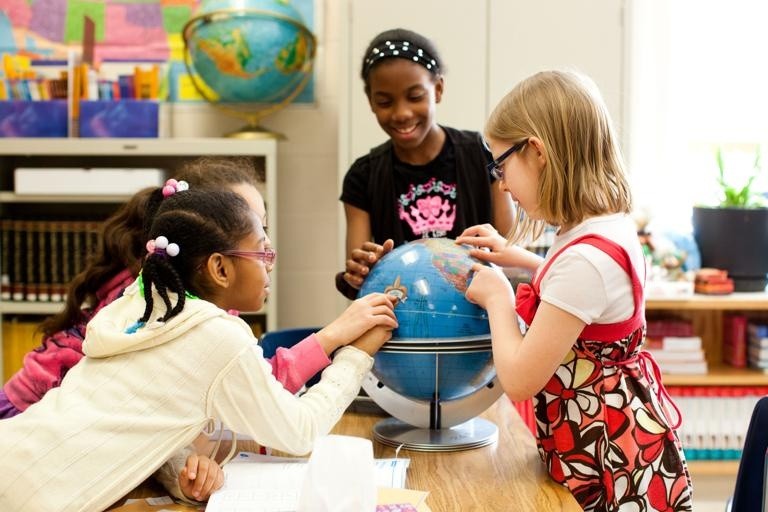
[355,237,525,452]
[182,0,316,141]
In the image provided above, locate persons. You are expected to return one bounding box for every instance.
[455,70,693,512]
[0,188,400,511]
[337,28,517,291]
[0,157,401,441]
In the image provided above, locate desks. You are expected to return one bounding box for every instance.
[104,393,584,512]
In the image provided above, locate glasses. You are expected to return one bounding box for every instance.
[195,247,276,275]
[486,137,530,181]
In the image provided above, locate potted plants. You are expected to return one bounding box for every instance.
[692,141,768,292]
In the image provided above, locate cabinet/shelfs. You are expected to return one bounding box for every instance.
[644,292,768,460]
[0,138,278,387]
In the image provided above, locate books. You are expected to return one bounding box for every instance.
[641,310,768,377]
[0,220,111,302]
[202,432,430,510]
[3,76,133,100]
